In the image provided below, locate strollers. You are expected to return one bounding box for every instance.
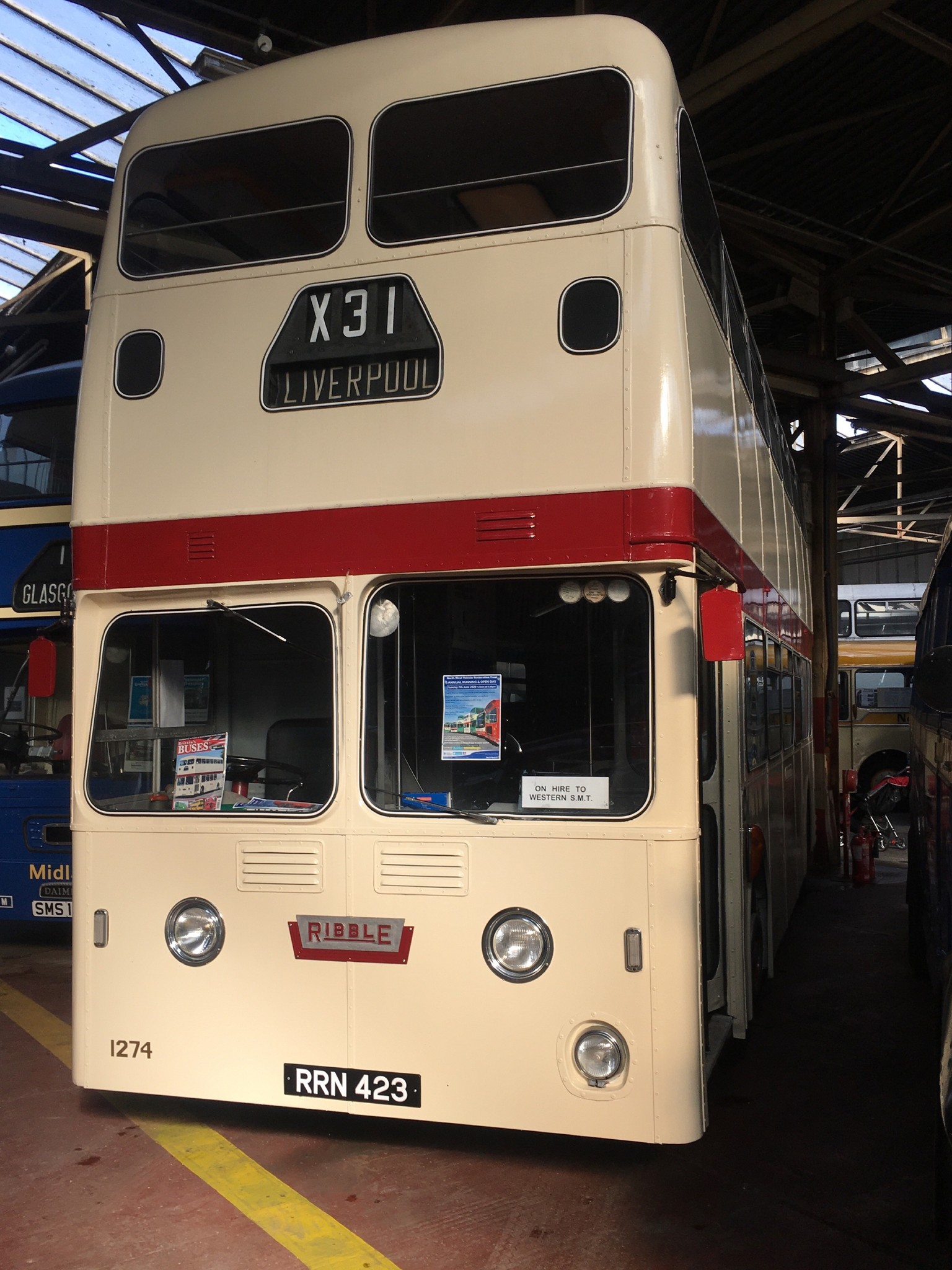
[839,764,909,852]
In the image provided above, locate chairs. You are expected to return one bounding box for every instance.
[48,712,107,776]
[265,718,335,803]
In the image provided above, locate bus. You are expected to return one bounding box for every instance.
[0,362,82,939]
[837,592,928,824]
[67,12,821,1144]
[911,514,951,929]
